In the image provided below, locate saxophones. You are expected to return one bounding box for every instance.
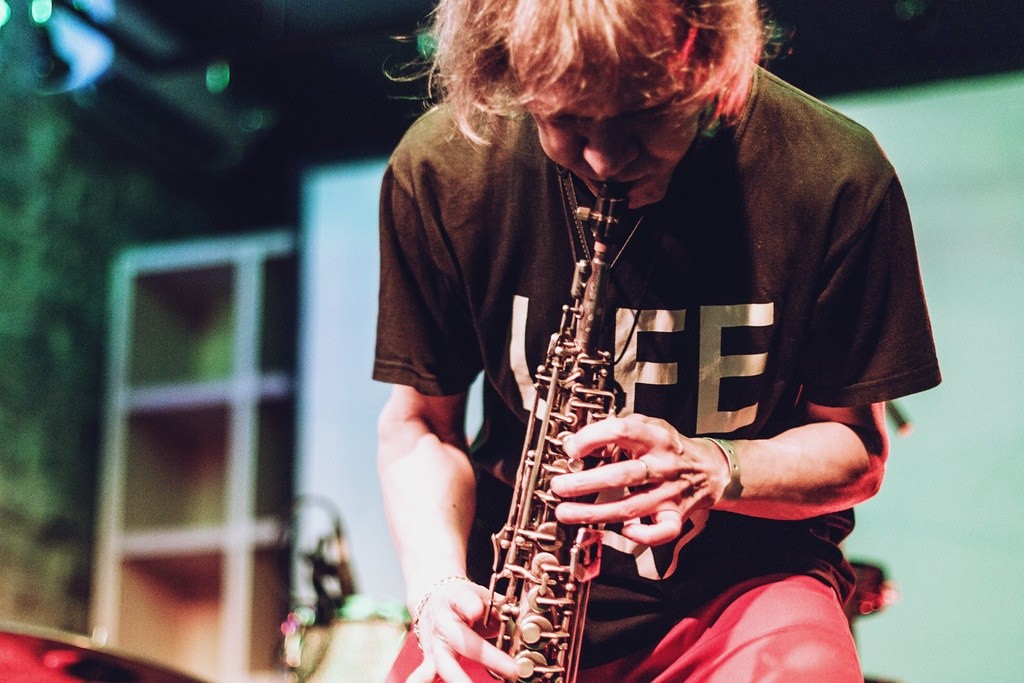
[484,181,635,683]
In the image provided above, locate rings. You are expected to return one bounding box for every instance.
[638,458,650,486]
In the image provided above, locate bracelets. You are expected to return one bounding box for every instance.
[703,436,741,497]
[412,576,469,656]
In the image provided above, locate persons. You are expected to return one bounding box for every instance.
[371,0,942,683]
